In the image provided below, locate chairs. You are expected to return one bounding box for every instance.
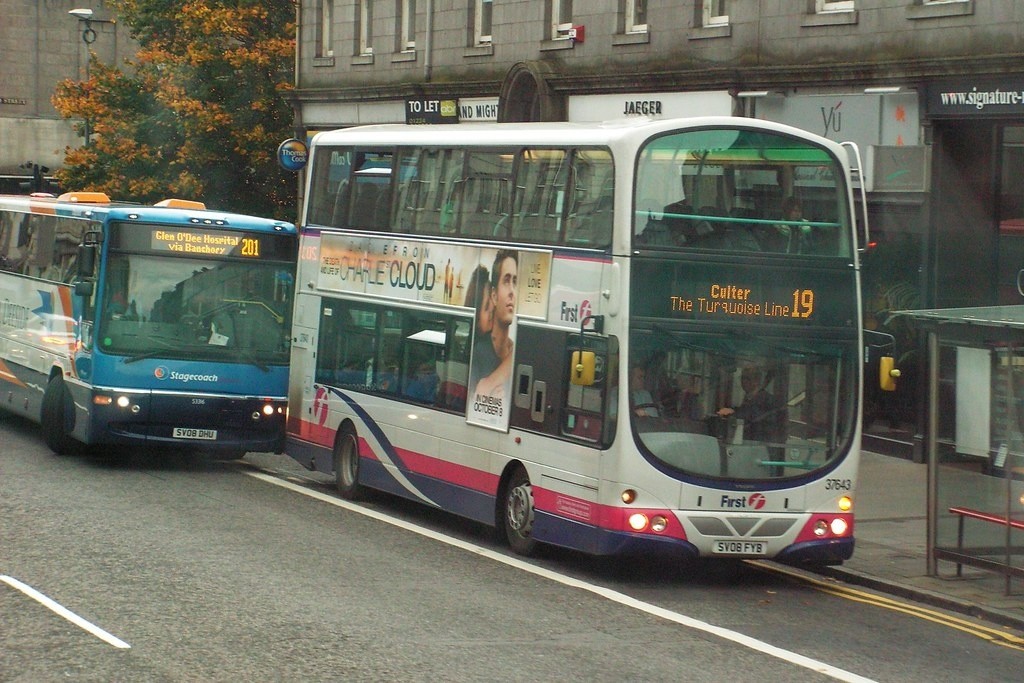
[325,171,826,447]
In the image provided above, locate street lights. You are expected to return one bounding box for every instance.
[68,7,96,171]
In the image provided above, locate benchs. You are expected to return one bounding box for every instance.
[942,505,1024,577]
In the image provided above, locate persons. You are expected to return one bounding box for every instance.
[760,196,829,256]
[438,180,478,237]
[468,249,520,405]
[377,360,403,395]
[465,264,515,405]
[713,364,781,477]
[348,182,380,230]
[639,344,680,422]
[334,356,367,388]
[609,364,659,422]
[405,357,441,406]
[178,284,220,326]
[864,331,909,433]
[570,195,613,245]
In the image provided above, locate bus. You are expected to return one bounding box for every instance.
[1,191,300,470]
[286,115,901,579]
